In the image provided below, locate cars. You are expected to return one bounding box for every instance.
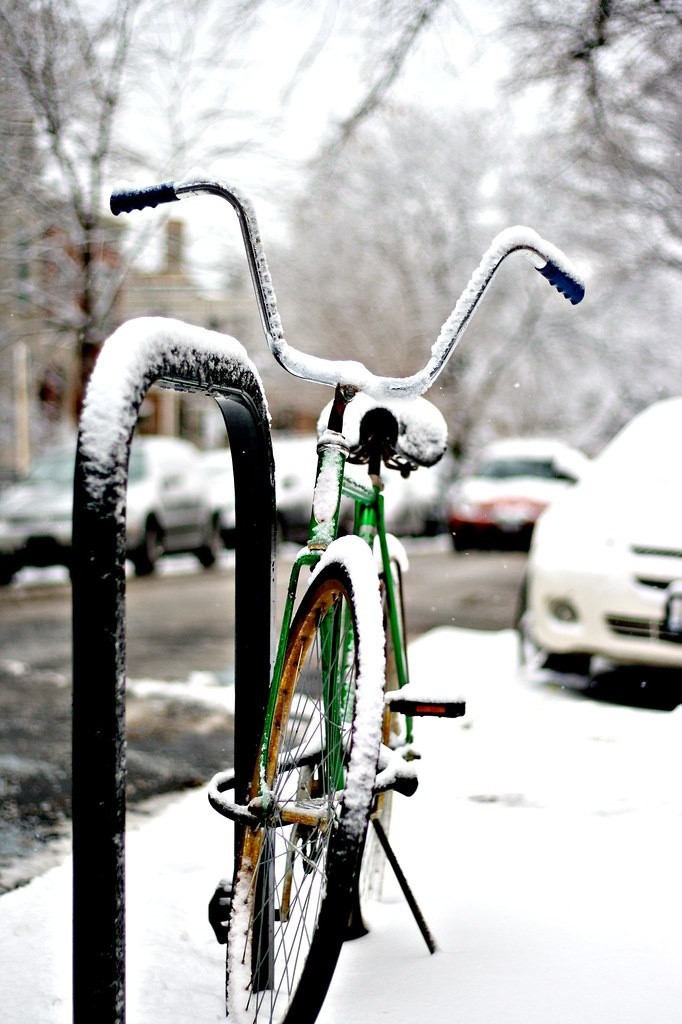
[0,433,458,588]
[447,436,580,554]
[516,394,682,684]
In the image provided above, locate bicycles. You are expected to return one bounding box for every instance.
[105,173,587,1023]
